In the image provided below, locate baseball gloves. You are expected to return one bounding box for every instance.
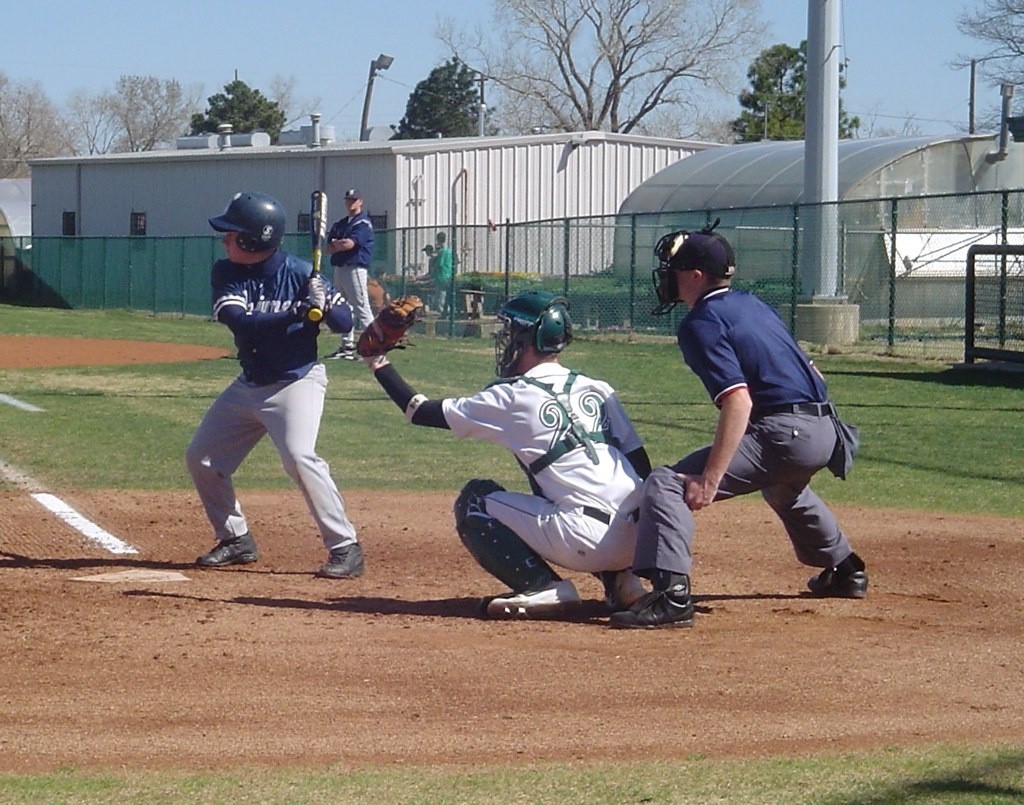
[356,294,423,357]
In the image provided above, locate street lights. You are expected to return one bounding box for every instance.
[361,52,395,141]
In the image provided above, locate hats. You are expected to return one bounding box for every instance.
[344,189,361,200]
[668,233,736,278]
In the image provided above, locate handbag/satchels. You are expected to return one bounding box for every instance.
[827,414,858,480]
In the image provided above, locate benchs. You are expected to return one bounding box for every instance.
[406,319,498,340]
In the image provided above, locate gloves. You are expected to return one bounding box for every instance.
[293,278,329,321]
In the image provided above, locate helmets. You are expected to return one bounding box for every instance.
[495,291,572,378]
[208,191,285,252]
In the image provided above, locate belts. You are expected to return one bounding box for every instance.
[750,403,834,416]
[584,506,640,526]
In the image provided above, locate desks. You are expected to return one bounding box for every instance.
[417,287,497,319]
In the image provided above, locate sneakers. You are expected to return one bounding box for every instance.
[319,544,365,578]
[601,570,653,609]
[196,531,258,566]
[808,566,868,598]
[487,578,582,621]
[609,590,696,628]
[323,347,356,360]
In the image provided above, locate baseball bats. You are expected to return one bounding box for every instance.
[307,190,328,322]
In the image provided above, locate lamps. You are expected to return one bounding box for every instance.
[571,137,585,149]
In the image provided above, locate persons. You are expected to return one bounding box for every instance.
[323,188,375,362]
[608,231,868,629]
[419,232,452,314]
[356,290,653,617]
[184,190,364,578]
